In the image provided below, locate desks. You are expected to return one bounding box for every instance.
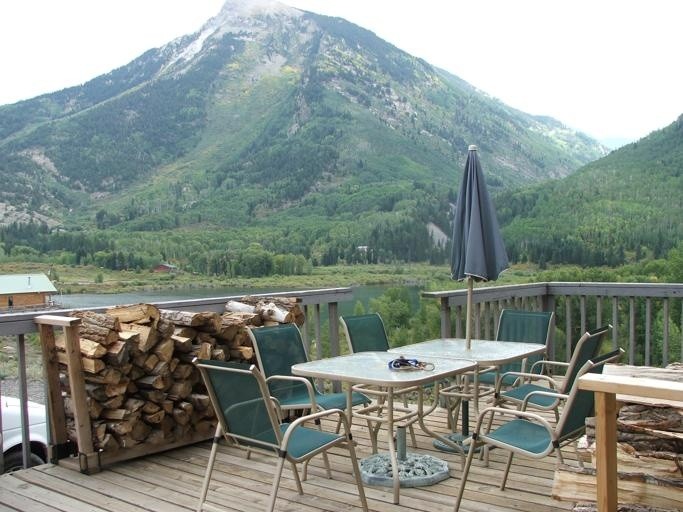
[388,334,547,454]
[291,350,478,489]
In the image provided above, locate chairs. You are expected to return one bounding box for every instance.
[451,308,560,437]
[244,323,378,479]
[454,346,631,511]
[337,314,454,449]
[193,357,373,511]
[476,324,614,462]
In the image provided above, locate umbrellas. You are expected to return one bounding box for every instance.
[433,145,513,454]
